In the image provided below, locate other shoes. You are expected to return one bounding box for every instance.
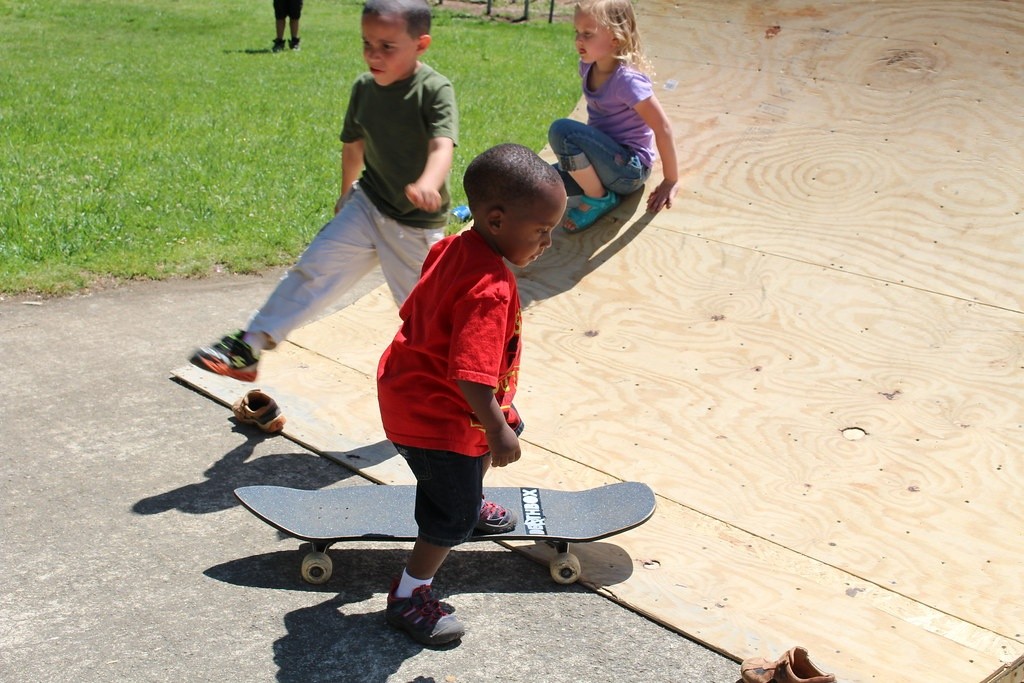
[288,36,300,52]
[273,38,285,53]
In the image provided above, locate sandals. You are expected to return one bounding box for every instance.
[562,188,620,234]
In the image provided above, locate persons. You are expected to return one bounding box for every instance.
[374,142,569,647]
[271,0,304,53]
[188,0,459,383]
[548,0,681,235]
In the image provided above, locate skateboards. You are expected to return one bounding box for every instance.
[231,479,658,585]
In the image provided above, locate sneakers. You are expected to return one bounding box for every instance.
[476,495,517,533]
[232,389,285,434]
[740,646,835,682]
[190,330,260,383]
[386,578,464,646]
